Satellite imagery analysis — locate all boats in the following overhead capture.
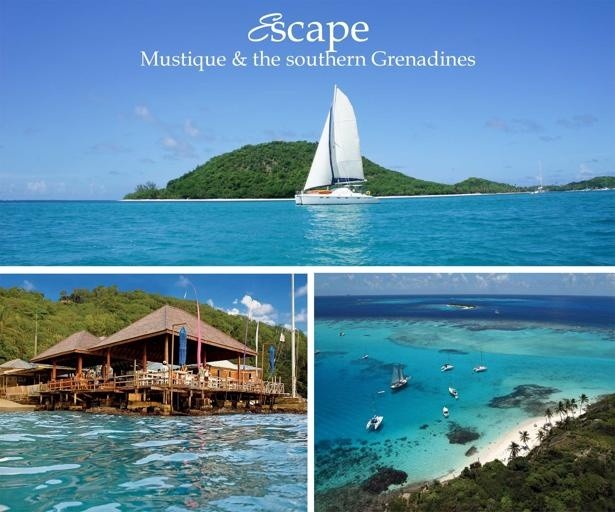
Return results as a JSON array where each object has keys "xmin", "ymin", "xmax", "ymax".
[{"xmin": 314, "ymin": 333, "xmax": 487, "ymax": 430}]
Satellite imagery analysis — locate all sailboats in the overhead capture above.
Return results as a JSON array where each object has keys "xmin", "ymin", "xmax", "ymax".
[{"xmin": 295, "ymin": 84, "xmax": 379, "ymax": 205}]
